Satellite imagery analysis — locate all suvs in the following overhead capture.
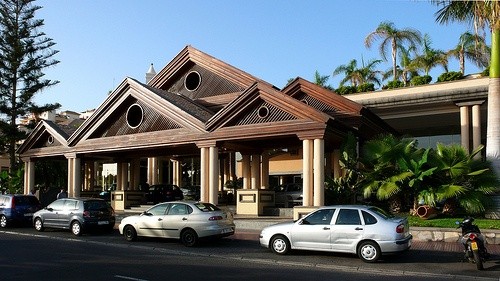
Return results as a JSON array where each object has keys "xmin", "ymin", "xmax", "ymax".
[
  {"xmin": 0, "ymin": 194, "xmax": 44, "ymax": 229},
  {"xmin": 32, "ymin": 197, "xmax": 116, "ymax": 236}
]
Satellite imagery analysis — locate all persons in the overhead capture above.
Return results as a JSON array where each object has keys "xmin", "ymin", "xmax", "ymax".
[
  {"xmin": 57, "ymin": 187, "xmax": 67, "ymax": 200},
  {"xmin": 0, "ymin": 189, "xmax": 7, "ymax": 196},
  {"xmin": 30, "ymin": 187, "xmax": 37, "ymax": 197}
]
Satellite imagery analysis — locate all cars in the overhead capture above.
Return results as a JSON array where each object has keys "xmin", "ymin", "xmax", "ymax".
[
  {"xmin": 259, "ymin": 204, "xmax": 413, "ymax": 263},
  {"xmin": 144, "ymin": 184, "xmax": 184, "ymax": 205},
  {"xmin": 118, "ymin": 200, "xmax": 236, "ymax": 248},
  {"xmin": 271, "ymin": 183, "xmax": 303, "ymax": 203},
  {"xmin": 99, "ymin": 184, "xmax": 117, "ymax": 200}
]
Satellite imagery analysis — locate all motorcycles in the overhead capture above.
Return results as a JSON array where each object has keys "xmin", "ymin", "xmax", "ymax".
[{"xmin": 455, "ymin": 216, "xmax": 488, "ymax": 270}]
